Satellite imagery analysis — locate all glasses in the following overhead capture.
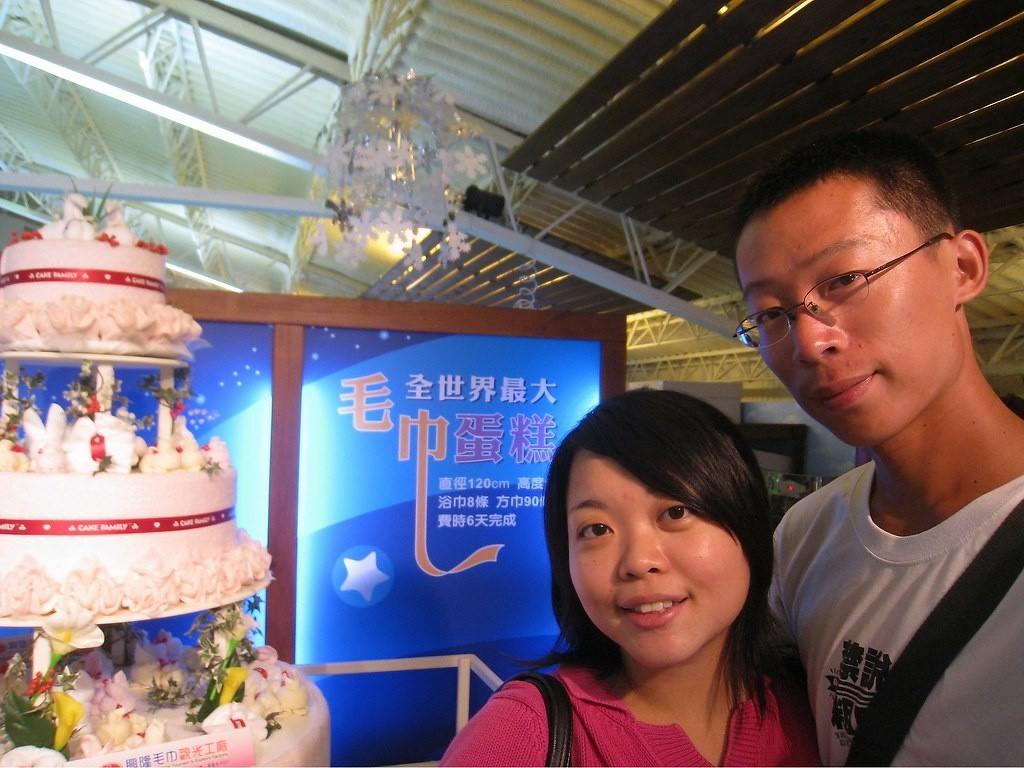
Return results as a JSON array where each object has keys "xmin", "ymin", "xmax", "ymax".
[{"xmin": 732, "ymin": 232, "xmax": 953, "ymax": 349}]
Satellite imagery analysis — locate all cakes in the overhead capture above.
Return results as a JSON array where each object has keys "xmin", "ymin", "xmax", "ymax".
[
  {"xmin": 0, "ymin": 598, "xmax": 331, "ymax": 768},
  {"xmin": 0, "ymin": 400, "xmax": 274, "ymax": 618},
  {"xmin": 0, "ymin": 180, "xmax": 203, "ymax": 360}
]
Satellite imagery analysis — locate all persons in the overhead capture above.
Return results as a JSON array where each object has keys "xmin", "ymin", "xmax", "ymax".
[
  {"xmin": 437, "ymin": 386, "xmax": 822, "ymax": 768},
  {"xmin": 732, "ymin": 141, "xmax": 1024, "ymax": 767}
]
